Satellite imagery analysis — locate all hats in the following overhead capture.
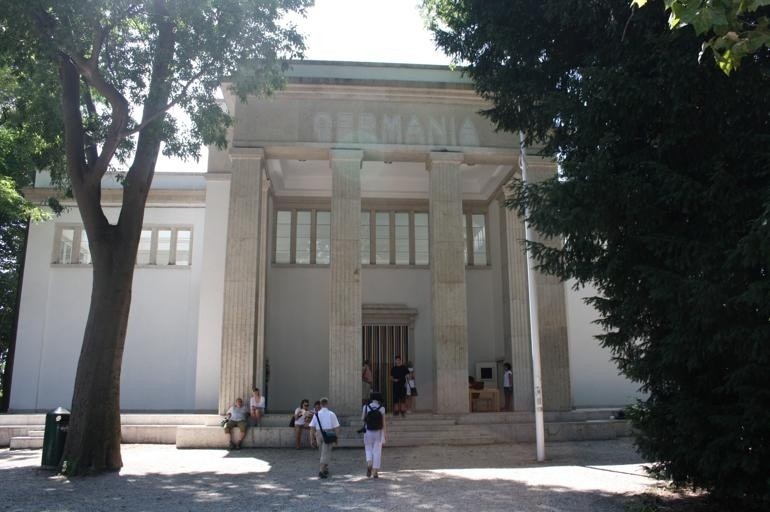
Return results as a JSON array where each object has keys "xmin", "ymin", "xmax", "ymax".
[{"xmin": 408, "ymin": 367, "xmax": 414, "ymax": 372}]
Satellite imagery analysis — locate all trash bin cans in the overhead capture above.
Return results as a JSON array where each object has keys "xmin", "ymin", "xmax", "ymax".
[{"xmin": 41, "ymin": 407, "xmax": 71, "ymax": 469}]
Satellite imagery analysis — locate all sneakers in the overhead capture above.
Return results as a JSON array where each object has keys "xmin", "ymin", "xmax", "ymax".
[
  {"xmin": 318, "ymin": 462, "xmax": 380, "ymax": 480},
  {"xmin": 229, "ymin": 443, "xmax": 241, "ymax": 450}
]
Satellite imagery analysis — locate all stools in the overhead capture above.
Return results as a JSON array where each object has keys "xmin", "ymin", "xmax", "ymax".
[{"xmin": 473, "ymin": 398, "xmax": 493, "ymax": 411}]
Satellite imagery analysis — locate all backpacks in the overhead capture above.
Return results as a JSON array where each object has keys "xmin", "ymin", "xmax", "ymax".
[{"xmin": 364, "ymin": 402, "xmax": 383, "ymax": 431}]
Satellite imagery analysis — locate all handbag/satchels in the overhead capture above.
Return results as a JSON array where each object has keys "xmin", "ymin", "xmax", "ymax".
[
  {"xmin": 410, "ymin": 387, "xmax": 417, "ymax": 396},
  {"xmin": 289, "ymin": 415, "xmax": 295, "ymax": 428},
  {"xmin": 323, "ymin": 429, "xmax": 337, "ymax": 445}
]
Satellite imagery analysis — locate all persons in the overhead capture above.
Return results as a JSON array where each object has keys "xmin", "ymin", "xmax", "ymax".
[
  {"xmin": 249, "ymin": 386, "xmax": 266, "ymax": 427},
  {"xmin": 310, "ymin": 396, "xmax": 341, "ymax": 478},
  {"xmin": 403, "ymin": 362, "xmax": 417, "ymax": 416},
  {"xmin": 308, "ymin": 400, "xmax": 320, "ymax": 450},
  {"xmin": 360, "ymin": 390, "xmax": 388, "ymax": 479},
  {"xmin": 388, "ymin": 354, "xmax": 409, "ymax": 418},
  {"xmin": 223, "ymin": 398, "xmax": 252, "ymax": 449},
  {"xmin": 609, "ymin": 410, "xmax": 626, "ymax": 420},
  {"xmin": 501, "ymin": 361, "xmax": 513, "ymax": 412},
  {"xmin": 363, "ymin": 359, "xmax": 374, "ymax": 404},
  {"xmin": 294, "ymin": 399, "xmax": 314, "ymax": 449}
]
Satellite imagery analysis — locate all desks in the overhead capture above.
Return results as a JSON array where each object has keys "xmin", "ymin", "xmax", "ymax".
[{"xmin": 469, "ymin": 386, "xmax": 501, "ymax": 412}]
